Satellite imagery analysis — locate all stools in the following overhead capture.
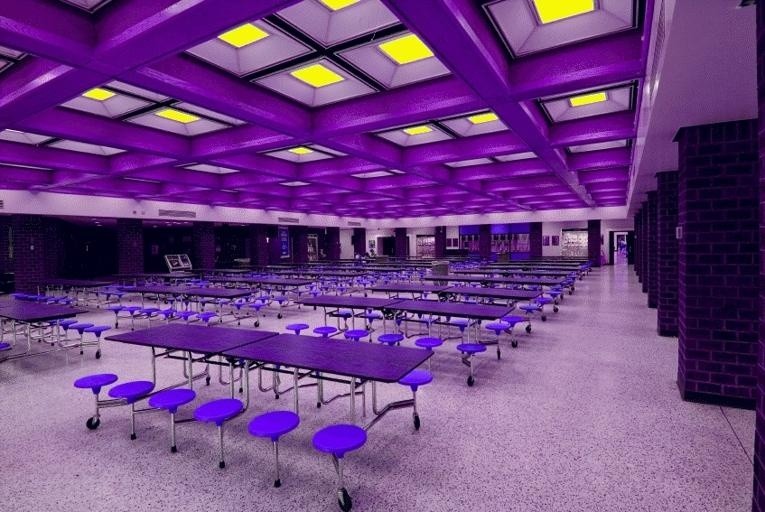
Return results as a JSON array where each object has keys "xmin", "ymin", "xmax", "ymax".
[
  {"xmin": 503, "ymin": 314, "xmax": 527, "ymax": 348},
  {"xmin": 107, "ymin": 380, "xmax": 155, "ymax": 441},
  {"xmin": 312, "ymin": 325, "xmax": 339, "ymax": 339},
  {"xmin": 10, "ymin": 257, "xmax": 314, "ymax": 327},
  {"xmin": 456, "ymin": 340, "xmax": 486, "ymax": 386},
  {"xmin": 396, "ymin": 370, "xmax": 431, "ymax": 431},
  {"xmin": 244, "ymin": 410, "xmax": 301, "ymax": 489},
  {"xmin": 193, "ymin": 396, "xmax": 244, "ymax": 471},
  {"xmin": 33, "ymin": 319, "xmax": 110, "ymax": 362},
  {"xmin": 147, "ymin": 386, "xmax": 196, "ymax": 454},
  {"xmin": 415, "ymin": 336, "xmax": 442, "ymax": 370},
  {"xmin": 311, "ymin": 424, "xmax": 368, "ymax": 511},
  {"xmin": 343, "ymin": 328, "xmax": 371, "ymax": 341},
  {"xmin": 377, "ymin": 333, "xmax": 406, "ymax": 347},
  {"xmin": 485, "ymin": 320, "xmax": 508, "ymax": 360},
  {"xmin": 301, "ymin": 255, "xmax": 596, "ymax": 333},
  {"xmin": 74, "ymin": 371, "xmax": 120, "ymax": 434},
  {"xmin": 285, "ymin": 322, "xmax": 311, "ymax": 334}
]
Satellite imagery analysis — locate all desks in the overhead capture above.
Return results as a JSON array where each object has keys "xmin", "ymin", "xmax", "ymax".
[
  {"xmin": 222, "ymin": 329, "xmax": 432, "ymax": 424},
  {"xmin": 0, "ymin": 297, "xmax": 91, "ymax": 355},
  {"xmin": 103, "ymin": 320, "xmax": 278, "ymax": 387}
]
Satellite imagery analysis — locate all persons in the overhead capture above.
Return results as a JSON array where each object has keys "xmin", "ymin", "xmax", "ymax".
[
  {"xmin": 620, "ymin": 237, "xmax": 627, "ymax": 256},
  {"xmin": 355, "ymin": 249, "xmax": 377, "ymax": 265}
]
